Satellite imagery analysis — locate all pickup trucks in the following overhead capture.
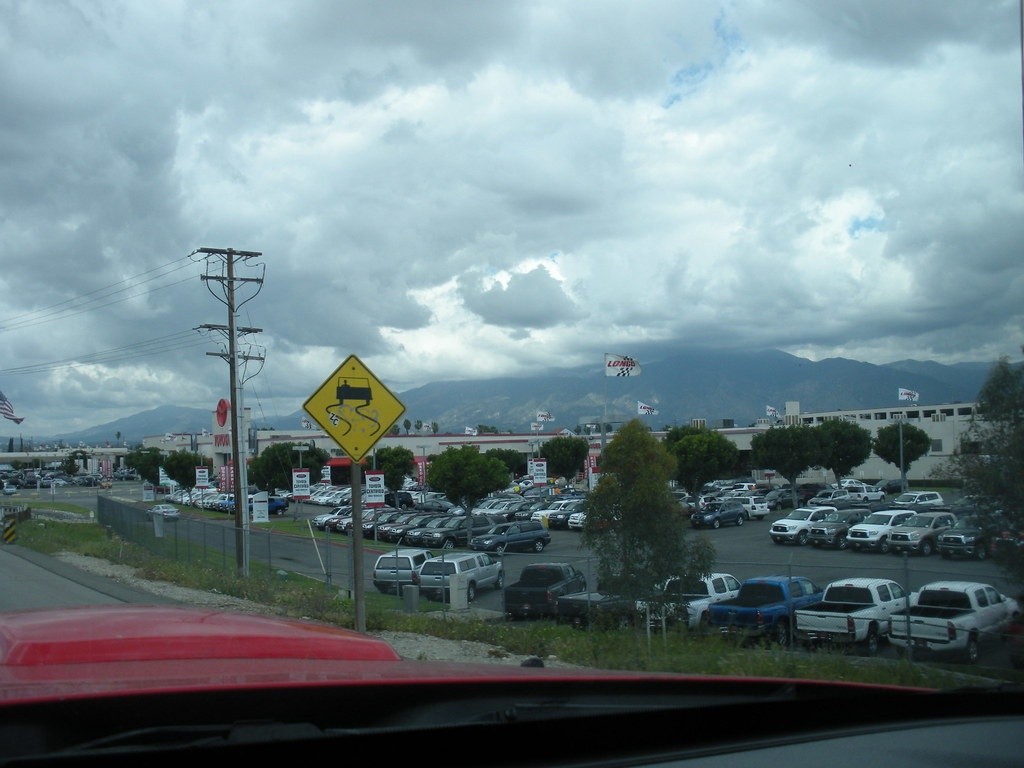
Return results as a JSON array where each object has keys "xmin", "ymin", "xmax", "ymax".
[
  {"xmin": 706, "ymin": 573, "xmax": 825, "ymax": 648},
  {"xmin": 793, "ymin": 576, "xmax": 919, "ymax": 658},
  {"xmin": 503, "ymin": 563, "xmax": 589, "ymax": 622},
  {"xmin": 553, "ymin": 563, "xmax": 680, "ymax": 633},
  {"xmin": 887, "ymin": 580, "xmax": 1023, "ymax": 665},
  {"xmin": 634, "ymin": 571, "xmax": 743, "ymax": 640}
]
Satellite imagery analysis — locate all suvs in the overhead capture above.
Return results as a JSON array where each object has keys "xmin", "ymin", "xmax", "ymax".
[
  {"xmin": 415, "ymin": 551, "xmax": 506, "ymax": 604},
  {"xmin": 371, "ymin": 548, "xmax": 435, "ymax": 596}
]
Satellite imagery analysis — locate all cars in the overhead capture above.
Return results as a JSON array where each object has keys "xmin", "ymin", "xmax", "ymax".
[
  {"xmin": 153, "ymin": 482, "xmax": 176, "ymax": 494},
  {"xmin": 2, "ymin": 485, "xmax": 17, "ymax": 495},
  {"xmin": 166, "ymin": 476, "xmax": 1024, "ymax": 563},
  {"xmin": 144, "ymin": 504, "xmax": 181, "ymax": 522},
  {"xmin": 9, "ymin": 462, "xmax": 140, "ymax": 489}
]
